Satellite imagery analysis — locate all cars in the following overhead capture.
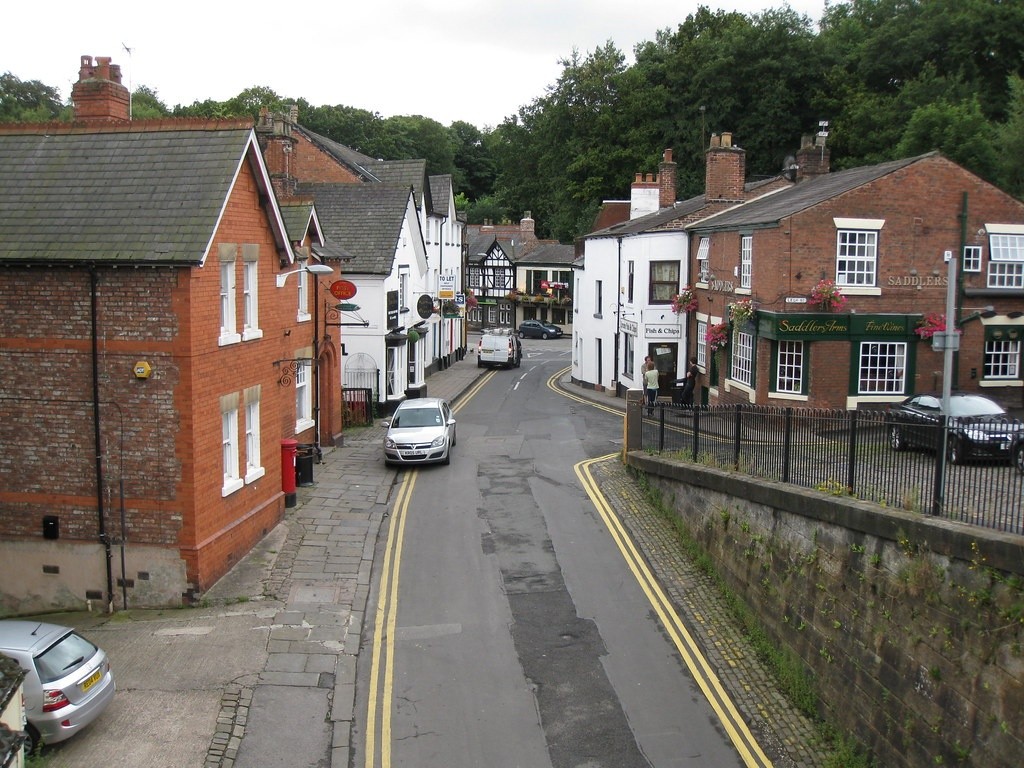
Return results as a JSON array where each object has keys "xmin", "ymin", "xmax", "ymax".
[
  {"xmin": 518, "ymin": 320, "xmax": 563, "ymax": 339},
  {"xmin": 1008, "ymin": 430, "xmax": 1024, "ymax": 475},
  {"xmin": 0, "ymin": 620, "xmax": 117, "ymax": 760},
  {"xmin": 886, "ymin": 389, "xmax": 1024, "ymax": 465},
  {"xmin": 380, "ymin": 397, "xmax": 456, "ymax": 466}
]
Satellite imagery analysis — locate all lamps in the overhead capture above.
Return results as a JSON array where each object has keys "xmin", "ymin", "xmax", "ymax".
[
  {"xmin": 276, "ymin": 265, "xmax": 334, "ymax": 288},
  {"xmin": 710, "ymin": 266, "xmax": 734, "ymax": 281},
  {"xmin": 704, "ymin": 266, "xmax": 724, "ymax": 281}
]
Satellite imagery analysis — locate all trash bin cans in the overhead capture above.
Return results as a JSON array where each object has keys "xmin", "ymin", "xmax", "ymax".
[
  {"xmin": 670, "ymin": 386, "xmax": 683, "ymax": 403},
  {"xmin": 296, "ymin": 443, "xmax": 314, "ymax": 488}
]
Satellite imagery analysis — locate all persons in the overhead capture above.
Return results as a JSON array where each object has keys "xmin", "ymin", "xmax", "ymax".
[
  {"xmin": 644, "ymin": 361, "xmax": 660, "ymax": 407},
  {"xmin": 681, "ymin": 357, "xmax": 698, "ymax": 408},
  {"xmin": 641, "ymin": 356, "xmax": 657, "ymax": 405}
]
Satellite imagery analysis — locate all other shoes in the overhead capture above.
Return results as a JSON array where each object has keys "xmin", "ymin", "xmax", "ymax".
[{"xmin": 648, "ymin": 413, "xmax": 653, "ymax": 416}]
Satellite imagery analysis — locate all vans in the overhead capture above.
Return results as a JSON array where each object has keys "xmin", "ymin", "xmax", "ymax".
[{"xmin": 476, "ymin": 329, "xmax": 522, "ymax": 368}]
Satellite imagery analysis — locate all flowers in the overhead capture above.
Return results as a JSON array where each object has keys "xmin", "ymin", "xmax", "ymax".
[
  {"xmin": 466, "ymin": 289, "xmax": 478, "ymax": 310},
  {"xmin": 810, "ymin": 278, "xmax": 849, "ymax": 312},
  {"xmin": 506, "ymin": 288, "xmax": 520, "ymax": 304},
  {"xmin": 443, "ymin": 299, "xmax": 460, "ymax": 314},
  {"xmin": 915, "ymin": 311, "xmax": 964, "ymax": 340},
  {"xmin": 705, "ymin": 322, "xmax": 728, "ymax": 373},
  {"xmin": 729, "ymin": 297, "xmax": 757, "ymax": 352},
  {"xmin": 672, "ymin": 285, "xmax": 699, "ymax": 316},
  {"xmin": 548, "ymin": 294, "xmax": 557, "ymax": 302}
]
